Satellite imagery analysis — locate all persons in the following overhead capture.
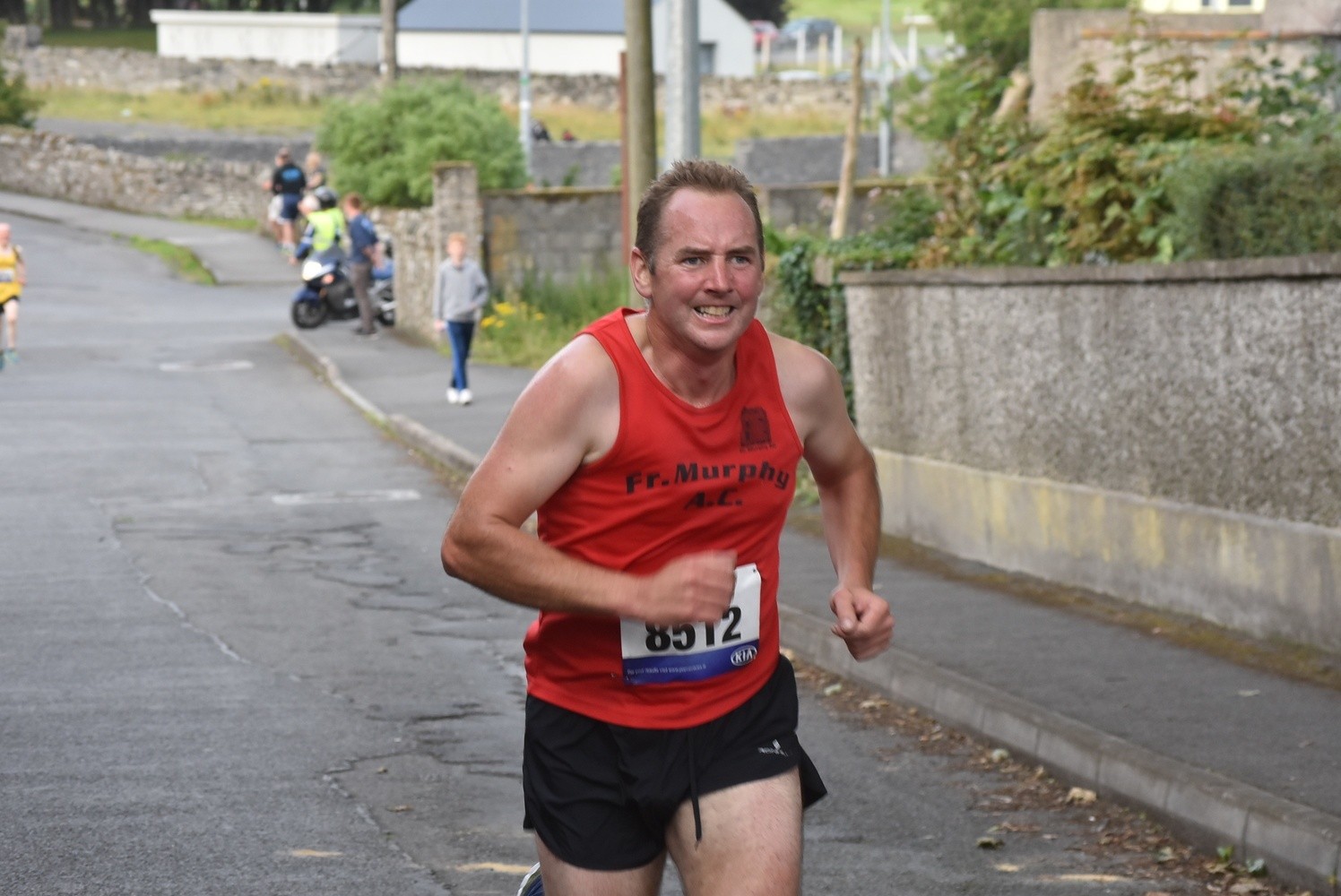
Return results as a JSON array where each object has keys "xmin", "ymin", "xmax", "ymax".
[
  {"xmin": 441, "ymin": 159, "xmax": 896, "ymax": 896},
  {"xmin": 0, "ymin": 222, "xmax": 27, "ymax": 355},
  {"xmin": 261, "ymin": 147, "xmax": 389, "ymax": 335},
  {"xmin": 434, "ymin": 232, "xmax": 489, "ymax": 408}
]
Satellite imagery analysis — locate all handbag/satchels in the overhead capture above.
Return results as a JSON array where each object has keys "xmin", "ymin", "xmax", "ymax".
[{"xmin": 267, "ymin": 194, "xmax": 284, "ymax": 220}]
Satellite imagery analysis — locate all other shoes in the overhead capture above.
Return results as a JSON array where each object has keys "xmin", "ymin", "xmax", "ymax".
[
  {"xmin": 459, "ymin": 389, "xmax": 473, "ymax": 405},
  {"xmin": 446, "ymin": 387, "xmax": 460, "ymax": 403}
]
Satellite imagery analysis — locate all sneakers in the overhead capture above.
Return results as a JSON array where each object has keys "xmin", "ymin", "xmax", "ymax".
[{"xmin": 517, "ymin": 861, "xmax": 546, "ymax": 896}]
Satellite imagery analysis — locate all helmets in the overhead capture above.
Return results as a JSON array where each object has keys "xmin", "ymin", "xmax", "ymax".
[{"xmin": 315, "ymin": 188, "xmax": 336, "ymax": 209}]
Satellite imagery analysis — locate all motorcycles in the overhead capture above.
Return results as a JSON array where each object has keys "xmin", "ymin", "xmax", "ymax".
[{"xmin": 290, "ymin": 243, "xmax": 395, "ymax": 330}]
